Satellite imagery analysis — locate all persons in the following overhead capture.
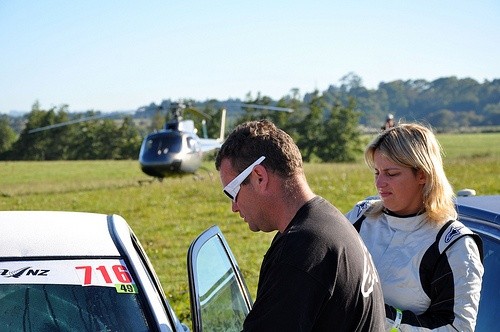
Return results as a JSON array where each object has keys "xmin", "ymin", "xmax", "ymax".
[
  {"xmin": 381, "ymin": 114, "xmax": 397, "ymax": 130},
  {"xmin": 215, "ymin": 120, "xmax": 387, "ymax": 332},
  {"xmin": 343, "ymin": 124, "xmax": 484, "ymax": 332}
]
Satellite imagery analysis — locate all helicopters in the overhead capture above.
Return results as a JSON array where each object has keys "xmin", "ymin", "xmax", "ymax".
[{"xmin": 27, "ymin": 99, "xmax": 294, "ymax": 184}]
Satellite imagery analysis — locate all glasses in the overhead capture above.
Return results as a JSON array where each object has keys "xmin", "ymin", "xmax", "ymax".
[{"xmin": 223, "ymin": 156, "xmax": 267, "ymax": 203}]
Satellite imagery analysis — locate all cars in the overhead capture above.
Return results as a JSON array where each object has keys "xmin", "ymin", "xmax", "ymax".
[
  {"xmin": 0, "ymin": 211, "xmax": 254, "ymax": 332},
  {"xmin": 369, "ymin": 195, "xmax": 500, "ymax": 332}
]
